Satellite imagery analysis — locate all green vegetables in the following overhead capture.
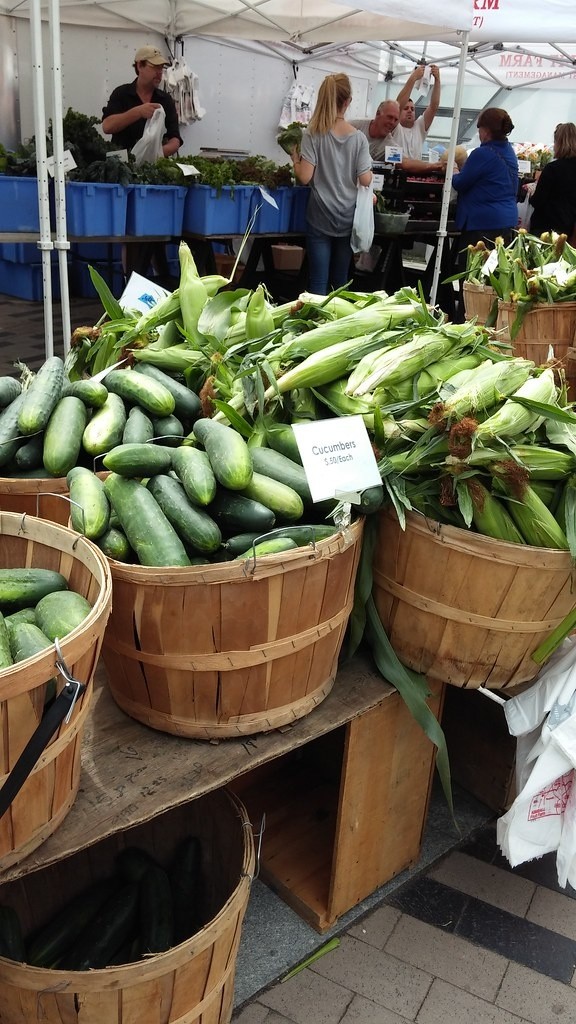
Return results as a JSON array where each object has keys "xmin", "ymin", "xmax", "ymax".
[{"xmin": 0, "ymin": 107, "xmax": 308, "ymax": 200}]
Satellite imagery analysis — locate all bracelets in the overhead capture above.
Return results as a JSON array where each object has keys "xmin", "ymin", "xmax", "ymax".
[{"xmin": 294, "ymin": 160, "xmax": 300, "ymax": 167}]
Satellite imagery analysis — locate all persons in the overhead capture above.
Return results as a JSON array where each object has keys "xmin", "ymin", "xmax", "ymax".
[
  {"xmin": 395, "ymin": 65, "xmax": 441, "ymax": 160},
  {"xmin": 347, "ymin": 99, "xmax": 447, "ymax": 273},
  {"xmin": 103, "ymin": 46, "xmax": 184, "ymax": 287},
  {"xmin": 528, "ymin": 123, "xmax": 576, "ymax": 247},
  {"xmin": 451, "ymin": 107, "xmax": 519, "ymax": 325},
  {"xmin": 289, "ymin": 73, "xmax": 373, "ymax": 301}
]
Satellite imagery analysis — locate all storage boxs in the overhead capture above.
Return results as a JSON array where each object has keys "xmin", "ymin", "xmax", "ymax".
[{"xmin": 0, "ymin": 175, "xmax": 311, "ymax": 302}]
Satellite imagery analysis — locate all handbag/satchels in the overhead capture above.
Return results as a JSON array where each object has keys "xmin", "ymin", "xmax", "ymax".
[
  {"xmin": 517, "ymin": 186, "xmax": 527, "ymax": 203},
  {"xmin": 515, "ymin": 191, "xmax": 534, "ymax": 234},
  {"xmin": 350, "ymin": 172, "xmax": 375, "ymax": 254}
]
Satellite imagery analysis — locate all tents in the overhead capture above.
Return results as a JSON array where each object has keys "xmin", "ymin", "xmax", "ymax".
[{"xmin": 1, "ymin": 0, "xmax": 576, "ymax": 361}]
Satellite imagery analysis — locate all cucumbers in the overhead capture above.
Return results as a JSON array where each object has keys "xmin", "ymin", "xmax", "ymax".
[{"xmin": 0, "ymin": 356, "xmax": 383, "ymax": 670}]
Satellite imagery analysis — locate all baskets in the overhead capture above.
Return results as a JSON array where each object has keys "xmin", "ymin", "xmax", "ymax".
[
  {"xmin": 375, "ymin": 501, "xmax": 576, "ymax": 691},
  {"xmin": 463, "ymin": 276, "xmax": 575, "ymax": 403},
  {"xmin": 2, "ymin": 470, "xmax": 118, "ymax": 528},
  {"xmin": 109, "ymin": 505, "xmax": 365, "ymax": 741},
  {"xmin": 1, "ymin": 515, "xmax": 113, "ymax": 867},
  {"xmin": 1, "ymin": 790, "xmax": 257, "ymax": 1024}
]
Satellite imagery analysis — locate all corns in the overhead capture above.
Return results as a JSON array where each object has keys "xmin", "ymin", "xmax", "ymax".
[{"xmin": 65, "ymin": 228, "xmax": 576, "ymax": 563}]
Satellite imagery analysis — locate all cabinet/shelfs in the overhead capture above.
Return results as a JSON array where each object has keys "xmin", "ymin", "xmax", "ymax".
[
  {"xmin": 233, "ymin": 673, "xmax": 448, "ymax": 935},
  {"xmin": 375, "ymin": 166, "xmax": 457, "ymax": 236}
]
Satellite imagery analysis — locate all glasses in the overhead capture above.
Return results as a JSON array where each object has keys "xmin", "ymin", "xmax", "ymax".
[{"xmin": 143, "ymin": 63, "xmax": 167, "ymax": 72}]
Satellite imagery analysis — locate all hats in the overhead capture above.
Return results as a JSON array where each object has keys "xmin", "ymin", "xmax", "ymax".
[
  {"xmin": 477, "ymin": 108, "xmax": 515, "ymax": 133},
  {"xmin": 134, "ymin": 46, "xmax": 172, "ymax": 67}
]
